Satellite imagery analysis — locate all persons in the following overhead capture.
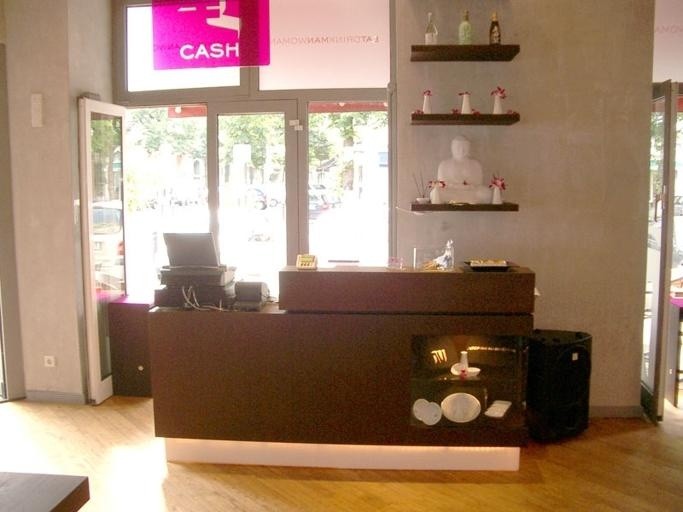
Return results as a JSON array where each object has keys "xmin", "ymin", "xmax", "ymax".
[{"xmin": 430, "ymin": 135, "xmax": 491, "ymax": 205}]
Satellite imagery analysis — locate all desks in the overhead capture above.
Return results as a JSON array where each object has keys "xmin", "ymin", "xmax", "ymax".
[{"xmin": 0, "ymin": 472, "xmax": 89, "ymax": 512}]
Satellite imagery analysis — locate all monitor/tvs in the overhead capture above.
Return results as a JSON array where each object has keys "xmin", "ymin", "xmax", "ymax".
[{"xmin": 162, "ymin": 232, "xmax": 218, "ymax": 267}]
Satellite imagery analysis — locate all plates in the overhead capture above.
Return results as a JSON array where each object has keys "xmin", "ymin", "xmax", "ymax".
[{"xmin": 470, "ymin": 256, "xmax": 509, "ymax": 272}]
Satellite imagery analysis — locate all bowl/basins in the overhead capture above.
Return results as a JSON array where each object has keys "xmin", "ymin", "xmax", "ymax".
[
  {"xmin": 467, "ymin": 367, "xmax": 480, "ymax": 376},
  {"xmin": 416, "ymin": 196, "xmax": 429, "ymax": 203},
  {"xmin": 450, "ymin": 362, "xmax": 460, "ymax": 375},
  {"xmin": 413, "ymin": 392, "xmax": 481, "ymax": 427}
]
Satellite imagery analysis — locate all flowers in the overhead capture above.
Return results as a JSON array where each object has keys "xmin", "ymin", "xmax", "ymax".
[
  {"xmin": 489, "ymin": 87, "xmax": 507, "ymax": 99},
  {"xmin": 489, "ymin": 175, "xmax": 506, "ymax": 191},
  {"xmin": 423, "ymin": 89, "xmax": 431, "ymax": 94},
  {"xmin": 458, "ymin": 90, "xmax": 471, "ymax": 95},
  {"xmin": 426, "ymin": 180, "xmax": 446, "ymax": 188}
]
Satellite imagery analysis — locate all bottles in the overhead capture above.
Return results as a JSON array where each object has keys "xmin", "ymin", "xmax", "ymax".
[
  {"xmin": 458, "ymin": 9, "xmax": 472, "ymax": 44},
  {"xmin": 489, "ymin": 10, "xmax": 501, "ymax": 45},
  {"xmin": 460, "ymin": 351, "xmax": 468, "ymax": 373},
  {"xmin": 425, "ymin": 12, "xmax": 439, "ymax": 44}
]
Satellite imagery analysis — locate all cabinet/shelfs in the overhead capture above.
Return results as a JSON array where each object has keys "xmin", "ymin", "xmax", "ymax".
[{"xmin": 411, "ymin": 44, "xmax": 521, "ymax": 212}]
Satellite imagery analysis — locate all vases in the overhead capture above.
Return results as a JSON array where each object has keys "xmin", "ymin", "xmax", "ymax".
[
  {"xmin": 461, "ymin": 96, "xmax": 471, "ymax": 115},
  {"xmin": 492, "ymin": 188, "xmax": 502, "ymax": 205},
  {"xmin": 492, "ymin": 97, "xmax": 503, "ymax": 114},
  {"xmin": 423, "ymin": 95, "xmax": 432, "ymax": 115},
  {"xmin": 430, "ymin": 188, "xmax": 440, "ymax": 204}
]
